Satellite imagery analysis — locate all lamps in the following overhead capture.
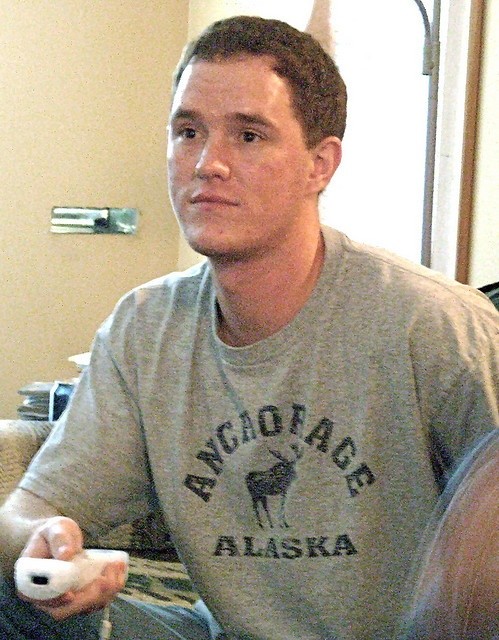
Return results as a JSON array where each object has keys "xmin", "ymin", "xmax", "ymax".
[{"xmin": 302, "ymin": 0, "xmax": 442, "ymax": 269}]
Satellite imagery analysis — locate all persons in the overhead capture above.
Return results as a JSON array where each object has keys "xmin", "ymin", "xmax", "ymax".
[{"xmin": 2, "ymin": 15, "xmax": 497, "ymax": 640}]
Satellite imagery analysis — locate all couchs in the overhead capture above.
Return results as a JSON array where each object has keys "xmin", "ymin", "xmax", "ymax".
[{"xmin": 0, "ymin": 420, "xmax": 222, "ymax": 640}]
